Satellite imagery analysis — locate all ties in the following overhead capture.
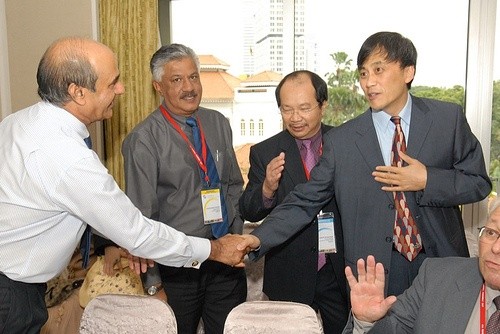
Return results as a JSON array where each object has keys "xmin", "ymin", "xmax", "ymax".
[
  {"xmin": 81, "ymin": 136, "xmax": 91, "ymax": 269},
  {"xmin": 303, "ymin": 139, "xmax": 326, "ymax": 272},
  {"xmin": 186, "ymin": 118, "xmax": 230, "ymax": 239},
  {"xmin": 485, "ymin": 295, "xmax": 500, "ymax": 334},
  {"xmin": 390, "ymin": 116, "xmax": 422, "ymax": 262}
]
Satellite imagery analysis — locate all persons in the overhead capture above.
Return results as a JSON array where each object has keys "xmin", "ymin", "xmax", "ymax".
[
  {"xmin": 237, "ymin": 69, "xmax": 349, "ymax": 333},
  {"xmin": 93, "ymin": 233, "xmax": 129, "ymax": 278},
  {"xmin": 120, "ymin": 43, "xmax": 246, "ymax": 334},
  {"xmin": 0, "ymin": 36, "xmax": 249, "ymax": 334},
  {"xmin": 233, "ymin": 31, "xmax": 493, "ymax": 317},
  {"xmin": 339, "ymin": 198, "xmax": 500, "ymax": 334}
]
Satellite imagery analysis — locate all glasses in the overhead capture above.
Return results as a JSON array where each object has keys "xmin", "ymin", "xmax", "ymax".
[
  {"xmin": 477, "ymin": 226, "xmax": 500, "ymax": 245},
  {"xmin": 280, "ymin": 103, "xmax": 319, "ymax": 114}
]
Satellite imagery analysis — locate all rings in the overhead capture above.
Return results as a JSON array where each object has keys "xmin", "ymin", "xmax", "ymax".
[{"xmin": 115, "ymin": 258, "xmax": 122, "ymax": 263}]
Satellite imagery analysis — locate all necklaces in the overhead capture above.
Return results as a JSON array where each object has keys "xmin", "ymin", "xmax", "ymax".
[{"xmin": 479, "ymin": 283, "xmax": 487, "ymax": 334}]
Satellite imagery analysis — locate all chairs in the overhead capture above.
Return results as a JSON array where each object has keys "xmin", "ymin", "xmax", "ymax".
[
  {"xmin": 79, "ymin": 293, "xmax": 177, "ymax": 334},
  {"xmin": 223, "ymin": 300, "xmax": 324, "ymax": 333}
]
